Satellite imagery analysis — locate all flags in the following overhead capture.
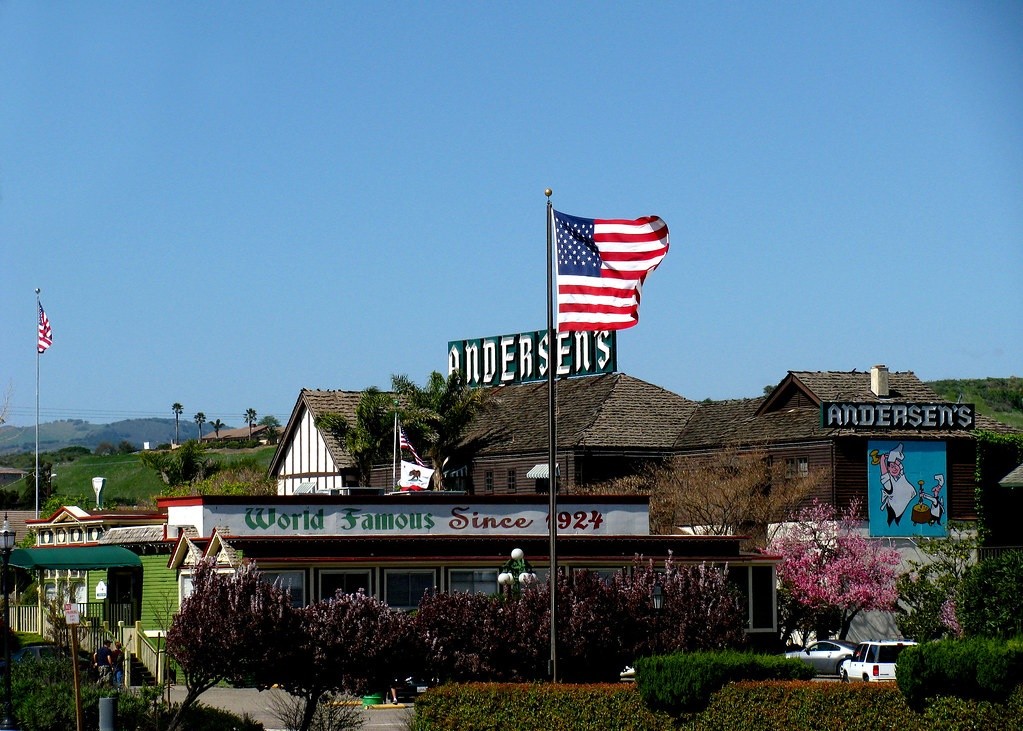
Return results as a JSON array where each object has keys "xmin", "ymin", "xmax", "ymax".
[
  {"xmin": 548, "ymin": 203, "xmax": 668, "ymax": 331},
  {"xmin": 400, "ymin": 426, "xmax": 424, "ymax": 467},
  {"xmin": 37, "ymin": 299, "xmax": 52, "ymax": 354}
]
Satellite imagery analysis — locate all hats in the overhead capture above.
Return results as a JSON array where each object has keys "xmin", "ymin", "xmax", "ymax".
[
  {"xmin": 116, "ymin": 642, "xmax": 121, "ymax": 647},
  {"xmin": 103, "ymin": 640, "xmax": 113, "ymax": 644}
]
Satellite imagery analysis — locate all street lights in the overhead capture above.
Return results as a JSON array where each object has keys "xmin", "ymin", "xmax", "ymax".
[
  {"xmin": 496, "ymin": 546, "xmax": 540, "ymax": 604},
  {"xmin": 0, "ymin": 511, "xmax": 29, "ymax": 731}
]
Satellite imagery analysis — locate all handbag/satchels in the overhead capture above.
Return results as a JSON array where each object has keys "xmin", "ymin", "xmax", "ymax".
[
  {"xmin": 109, "ymin": 671, "xmax": 113, "ymax": 685},
  {"xmin": 89, "ymin": 664, "xmax": 100, "ymax": 681},
  {"xmin": 116, "ymin": 652, "xmax": 124, "ymax": 668}
]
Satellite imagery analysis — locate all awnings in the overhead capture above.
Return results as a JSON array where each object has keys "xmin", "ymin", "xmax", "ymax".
[
  {"xmin": 526, "ymin": 463, "xmax": 559, "ymax": 479},
  {"xmin": 443, "ymin": 464, "xmax": 467, "ymax": 478}
]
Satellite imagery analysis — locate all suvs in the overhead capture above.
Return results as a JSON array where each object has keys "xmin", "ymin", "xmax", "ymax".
[{"xmin": 842, "ymin": 638, "xmax": 919, "ymax": 684}]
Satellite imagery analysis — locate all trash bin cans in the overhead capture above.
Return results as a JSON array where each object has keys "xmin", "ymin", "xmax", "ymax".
[{"xmin": 98, "ymin": 697, "xmax": 119, "ymax": 731}]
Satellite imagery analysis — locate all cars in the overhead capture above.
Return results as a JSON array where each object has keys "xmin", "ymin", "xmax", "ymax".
[
  {"xmin": 776, "ymin": 638, "xmax": 860, "ymax": 678},
  {"xmin": 0, "ymin": 644, "xmax": 90, "ymax": 684},
  {"xmin": 387, "ymin": 671, "xmax": 435, "ymax": 701}
]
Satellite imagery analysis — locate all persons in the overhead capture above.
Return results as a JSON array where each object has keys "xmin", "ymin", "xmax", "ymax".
[
  {"xmin": 93, "ymin": 640, "xmax": 124, "ymax": 688},
  {"xmin": 388, "ymin": 653, "xmax": 400, "ymax": 704}
]
